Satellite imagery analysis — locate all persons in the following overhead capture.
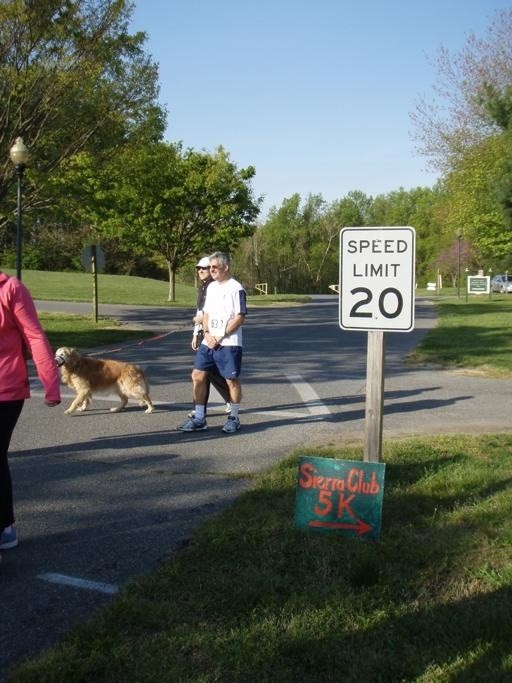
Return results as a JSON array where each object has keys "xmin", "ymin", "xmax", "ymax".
[
  {"xmin": 176, "ymin": 252, "xmax": 248, "ymax": 433},
  {"xmin": 187, "ymin": 256, "xmax": 232, "ymax": 418},
  {"xmin": 0, "ymin": 266, "xmax": 63, "ymax": 548}
]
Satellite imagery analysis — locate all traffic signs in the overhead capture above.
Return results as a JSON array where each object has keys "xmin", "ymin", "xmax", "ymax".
[{"xmin": 339, "ymin": 226, "xmax": 415, "ymax": 332}]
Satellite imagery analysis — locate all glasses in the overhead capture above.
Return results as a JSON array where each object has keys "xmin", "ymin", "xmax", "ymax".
[{"xmin": 198, "ymin": 267, "xmax": 207, "ymax": 270}]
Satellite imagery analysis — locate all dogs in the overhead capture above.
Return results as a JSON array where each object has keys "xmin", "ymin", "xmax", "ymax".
[{"xmin": 54, "ymin": 346, "xmax": 155, "ymax": 415}]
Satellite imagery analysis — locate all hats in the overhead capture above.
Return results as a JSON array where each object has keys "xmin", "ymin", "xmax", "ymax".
[{"xmin": 196, "ymin": 257, "xmax": 209, "ymax": 267}]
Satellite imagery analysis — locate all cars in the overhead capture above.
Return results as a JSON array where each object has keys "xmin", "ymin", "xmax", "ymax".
[{"xmin": 491, "ymin": 275, "xmax": 512, "ymax": 295}]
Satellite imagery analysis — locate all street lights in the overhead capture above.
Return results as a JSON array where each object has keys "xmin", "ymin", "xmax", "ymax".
[
  {"xmin": 9, "ymin": 137, "xmax": 26, "ymax": 283},
  {"xmin": 457, "ymin": 228, "xmax": 462, "ymax": 302}
]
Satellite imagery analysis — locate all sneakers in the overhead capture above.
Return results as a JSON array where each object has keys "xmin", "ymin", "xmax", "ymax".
[
  {"xmin": 0, "ymin": 525, "xmax": 17, "ymax": 549},
  {"xmin": 188, "ymin": 410, "xmax": 195, "ymax": 417},
  {"xmin": 178, "ymin": 418, "xmax": 208, "ymax": 432},
  {"xmin": 225, "ymin": 403, "xmax": 232, "ymax": 413},
  {"xmin": 222, "ymin": 416, "xmax": 241, "ymax": 432}
]
